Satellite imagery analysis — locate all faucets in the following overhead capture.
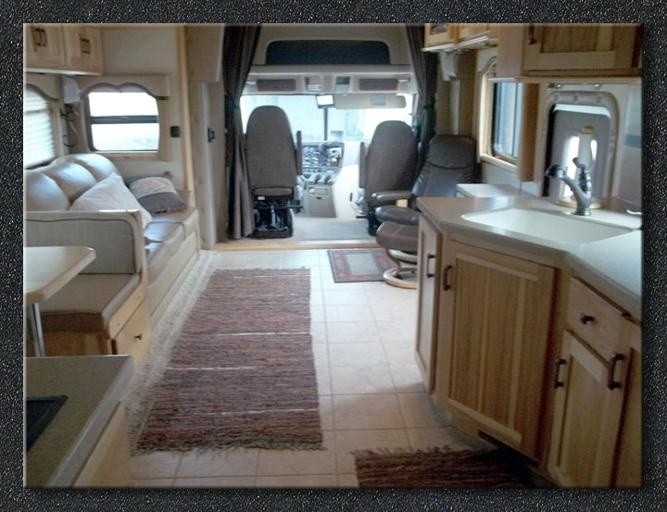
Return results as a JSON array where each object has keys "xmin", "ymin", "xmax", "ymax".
[{"xmin": 545, "ymin": 156, "xmax": 592, "ymax": 216}]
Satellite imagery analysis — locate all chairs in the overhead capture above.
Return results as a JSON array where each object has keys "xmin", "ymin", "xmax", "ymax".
[
  {"xmin": 241, "ymin": 105, "xmax": 303, "ymax": 239},
  {"xmin": 358, "ymin": 119, "xmax": 477, "ymax": 290}
]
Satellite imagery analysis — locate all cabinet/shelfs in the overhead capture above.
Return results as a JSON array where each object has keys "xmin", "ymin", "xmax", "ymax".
[
  {"xmin": 26, "ymin": 24, "xmax": 105, "ymax": 78},
  {"xmin": 422, "ymin": 24, "xmax": 498, "ymax": 48},
  {"xmin": 522, "ymin": 24, "xmax": 643, "ymax": 79},
  {"xmin": 26, "ymin": 282, "xmax": 151, "ymax": 370}
]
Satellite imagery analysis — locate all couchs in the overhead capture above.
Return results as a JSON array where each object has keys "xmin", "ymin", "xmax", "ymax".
[{"xmin": 22, "ymin": 154, "xmax": 202, "ymax": 265}]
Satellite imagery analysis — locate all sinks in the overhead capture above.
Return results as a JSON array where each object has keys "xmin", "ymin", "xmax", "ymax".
[{"xmin": 460, "ymin": 205, "xmax": 634, "ymax": 246}]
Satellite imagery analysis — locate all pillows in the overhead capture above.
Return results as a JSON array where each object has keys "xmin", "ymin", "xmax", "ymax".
[{"xmin": 69, "ymin": 171, "xmax": 187, "ymax": 230}]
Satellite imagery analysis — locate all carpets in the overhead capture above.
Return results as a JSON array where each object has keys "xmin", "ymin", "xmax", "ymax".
[
  {"xmin": 130, "ymin": 267, "xmax": 326, "ymax": 457},
  {"xmin": 327, "ymin": 243, "xmax": 385, "ymax": 282},
  {"xmin": 350, "ymin": 445, "xmax": 528, "ymax": 490}
]
